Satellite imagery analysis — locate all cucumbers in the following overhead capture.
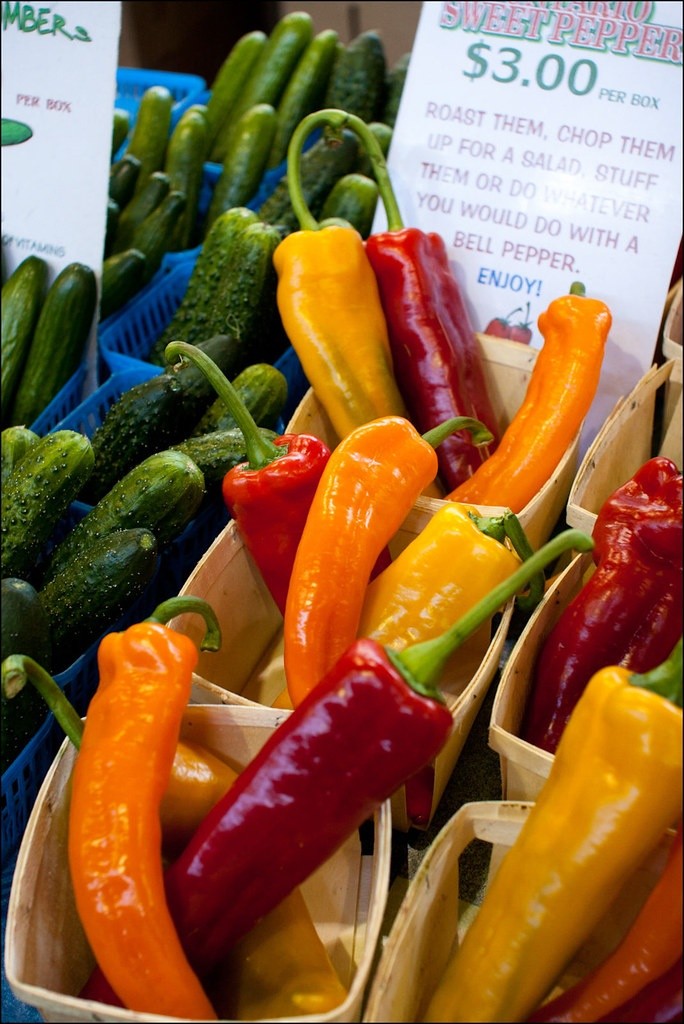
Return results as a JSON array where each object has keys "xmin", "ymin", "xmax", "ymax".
[{"xmin": 3, "ymin": 10, "xmax": 412, "ymax": 761}]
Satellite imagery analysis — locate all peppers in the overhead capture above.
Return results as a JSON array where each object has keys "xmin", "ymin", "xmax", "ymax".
[{"xmin": 1, "ymin": 109, "xmax": 684, "ymax": 1024}]
